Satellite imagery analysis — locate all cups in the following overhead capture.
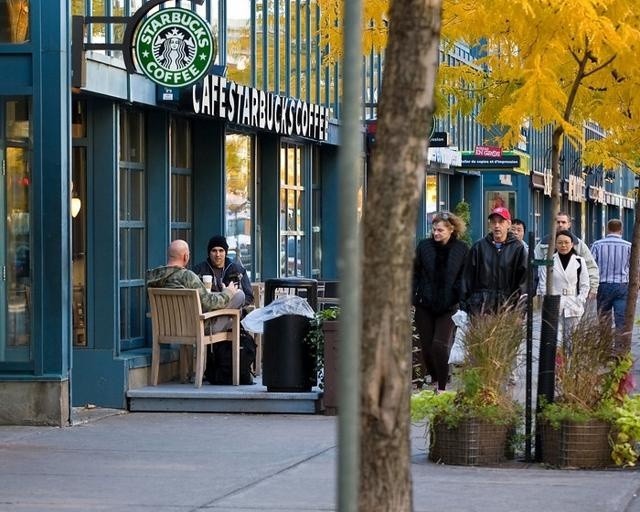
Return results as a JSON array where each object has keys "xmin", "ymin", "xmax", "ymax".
[{"xmin": 202, "ymin": 274, "xmax": 213, "ymax": 294}]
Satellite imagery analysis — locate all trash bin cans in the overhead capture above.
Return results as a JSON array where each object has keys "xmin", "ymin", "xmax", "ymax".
[{"xmin": 261, "ymin": 278, "xmax": 318, "ymax": 392}]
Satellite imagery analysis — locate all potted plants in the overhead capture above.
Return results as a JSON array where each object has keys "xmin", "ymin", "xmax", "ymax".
[
  {"xmin": 412, "ymin": 293, "xmax": 529, "ymax": 466},
  {"xmin": 538, "ymin": 303, "xmax": 640, "ymax": 470}
]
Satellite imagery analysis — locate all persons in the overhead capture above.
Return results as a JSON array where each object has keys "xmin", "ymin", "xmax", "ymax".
[
  {"xmin": 144, "ymin": 238, "xmax": 247, "ymax": 335},
  {"xmin": 507, "ymin": 218, "xmax": 529, "ymax": 386},
  {"xmin": 458, "ymin": 206, "xmax": 539, "ymax": 321},
  {"xmin": 192, "ymin": 235, "xmax": 256, "ymax": 381},
  {"xmin": 532, "ymin": 210, "xmax": 601, "ymax": 367},
  {"xmin": 589, "ymin": 218, "xmax": 633, "ymax": 358},
  {"xmin": 536, "ymin": 229, "xmax": 591, "ymax": 359},
  {"xmin": 409, "ymin": 210, "xmax": 472, "ymax": 390}
]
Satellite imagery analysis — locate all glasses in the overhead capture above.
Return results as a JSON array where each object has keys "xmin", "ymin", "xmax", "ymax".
[{"xmin": 437, "ymin": 211, "xmax": 453, "ymax": 224}]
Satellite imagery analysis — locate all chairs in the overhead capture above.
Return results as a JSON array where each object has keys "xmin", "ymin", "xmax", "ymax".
[{"xmin": 147, "ymin": 285, "xmax": 261, "ymax": 389}]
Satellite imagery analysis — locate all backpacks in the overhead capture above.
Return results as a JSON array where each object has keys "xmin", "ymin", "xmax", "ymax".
[{"xmin": 208, "ymin": 330, "xmax": 256, "ymax": 385}]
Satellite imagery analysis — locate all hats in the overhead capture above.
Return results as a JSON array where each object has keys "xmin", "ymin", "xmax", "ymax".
[
  {"xmin": 208, "ymin": 235, "xmax": 229, "ymax": 257},
  {"xmin": 488, "ymin": 207, "xmax": 511, "ymax": 221}
]
